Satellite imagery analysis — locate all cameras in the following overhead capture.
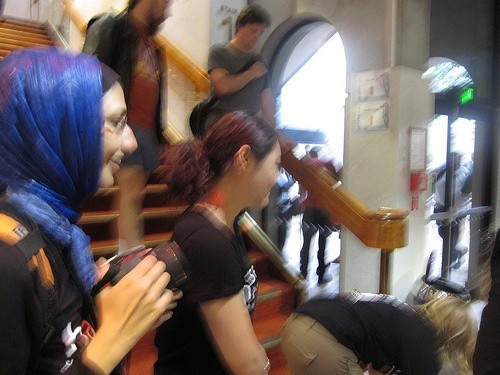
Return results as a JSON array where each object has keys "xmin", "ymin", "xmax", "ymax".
[{"xmin": 107, "ymin": 241, "xmax": 192, "ymax": 291}]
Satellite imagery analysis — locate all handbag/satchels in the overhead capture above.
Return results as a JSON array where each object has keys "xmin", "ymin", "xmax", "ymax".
[{"xmin": 189, "ymin": 97, "xmax": 208, "ymax": 140}]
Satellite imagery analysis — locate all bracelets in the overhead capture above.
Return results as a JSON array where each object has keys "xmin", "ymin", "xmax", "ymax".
[{"xmin": 264, "ymin": 359, "xmax": 270, "ymax": 372}]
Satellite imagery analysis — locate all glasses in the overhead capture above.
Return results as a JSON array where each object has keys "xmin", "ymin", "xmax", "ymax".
[{"xmin": 103, "ymin": 115, "xmax": 128, "ymax": 134}]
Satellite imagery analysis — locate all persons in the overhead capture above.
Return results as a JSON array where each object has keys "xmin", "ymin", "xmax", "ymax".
[
  {"xmin": 154, "ymin": 111, "xmax": 281, "ymax": 375},
  {"xmin": 0, "ymin": 46, "xmax": 172, "ymax": 375},
  {"xmin": 280, "ymin": 292, "xmax": 477, "ymax": 375},
  {"xmin": 1, "ymin": 0, "xmax": 500, "ymax": 375}
]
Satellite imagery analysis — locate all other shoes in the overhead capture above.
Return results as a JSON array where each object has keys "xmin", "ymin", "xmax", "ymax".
[{"xmin": 317, "ymin": 273, "xmax": 333, "ymax": 285}]
